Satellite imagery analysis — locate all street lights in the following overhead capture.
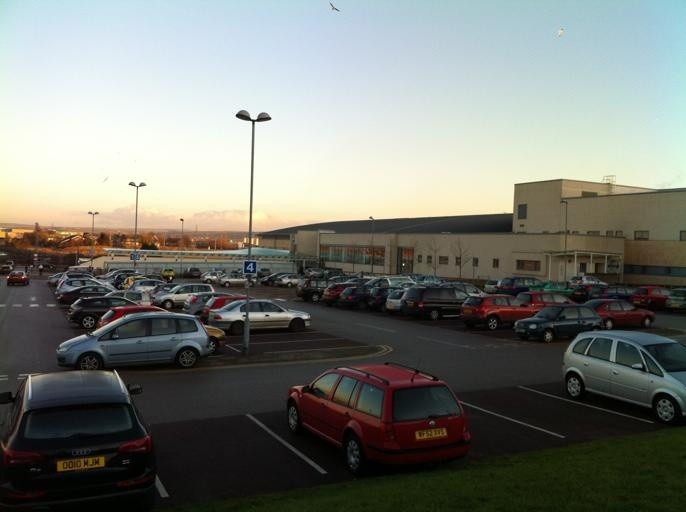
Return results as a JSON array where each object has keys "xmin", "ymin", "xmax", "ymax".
[
  {"xmin": 367, "ymin": 215, "xmax": 375, "ymax": 273},
  {"xmin": 559, "ymin": 199, "xmax": 570, "ymax": 283},
  {"xmin": 179, "ymin": 218, "xmax": 184, "ymax": 276},
  {"xmin": 234, "ymin": 109, "xmax": 272, "ymax": 354},
  {"xmin": 88, "ymin": 210, "xmax": 99, "ymax": 266},
  {"xmin": 128, "ymin": 180, "xmax": 147, "ymax": 285}
]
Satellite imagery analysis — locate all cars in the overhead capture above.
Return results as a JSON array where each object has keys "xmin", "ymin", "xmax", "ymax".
[
  {"xmin": 5, "ymin": 260, "xmax": 15, "ymax": 268},
  {"xmin": 561, "ymin": 327, "xmax": 686, "ymax": 427},
  {"xmin": 5, "ymin": 270, "xmax": 30, "ymax": 287},
  {"xmin": 0, "ymin": 265, "xmax": 12, "ymax": 274}
]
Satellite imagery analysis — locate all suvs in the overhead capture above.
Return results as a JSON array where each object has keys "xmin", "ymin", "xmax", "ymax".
[
  {"xmin": 0, "ymin": 366, "xmax": 160, "ymax": 511},
  {"xmin": 284, "ymin": 359, "xmax": 473, "ymax": 476}
]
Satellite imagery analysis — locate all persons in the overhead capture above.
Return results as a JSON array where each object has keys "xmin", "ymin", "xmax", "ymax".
[{"xmin": 39, "ymin": 264, "xmax": 44, "ymax": 276}]
